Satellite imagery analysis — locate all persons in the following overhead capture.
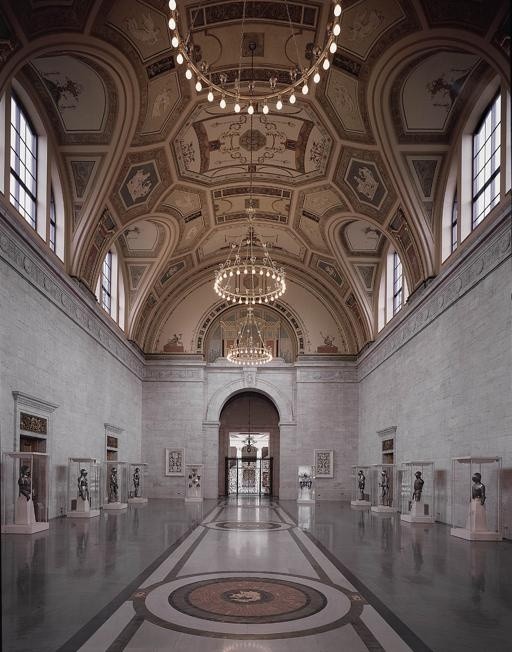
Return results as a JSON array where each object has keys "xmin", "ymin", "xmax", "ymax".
[
  {"xmin": 133, "ymin": 468, "xmax": 140, "ymax": 497},
  {"xmin": 378, "ymin": 470, "xmax": 390, "ymax": 506},
  {"xmin": 413, "ymin": 471, "xmax": 424, "ymax": 501},
  {"xmin": 78, "ymin": 468, "xmax": 89, "ymax": 501},
  {"xmin": 18, "ymin": 465, "xmax": 31, "ymax": 501},
  {"xmin": 109, "ymin": 468, "xmax": 118, "ymax": 502},
  {"xmin": 471, "ymin": 473, "xmax": 485, "ymax": 505},
  {"xmin": 358, "ymin": 470, "xmax": 365, "ymax": 499}
]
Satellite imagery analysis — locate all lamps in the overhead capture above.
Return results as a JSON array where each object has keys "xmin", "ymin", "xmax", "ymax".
[
  {"xmin": 211, "ymin": 201, "xmax": 288, "ymax": 369},
  {"xmin": 166, "ymin": 1, "xmax": 345, "ymax": 115}
]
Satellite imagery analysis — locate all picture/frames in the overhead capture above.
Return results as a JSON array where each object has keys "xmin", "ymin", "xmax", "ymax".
[
  {"xmin": 165, "ymin": 447, "xmax": 186, "ymax": 477},
  {"xmin": 314, "ymin": 448, "xmax": 334, "ymax": 478}
]
